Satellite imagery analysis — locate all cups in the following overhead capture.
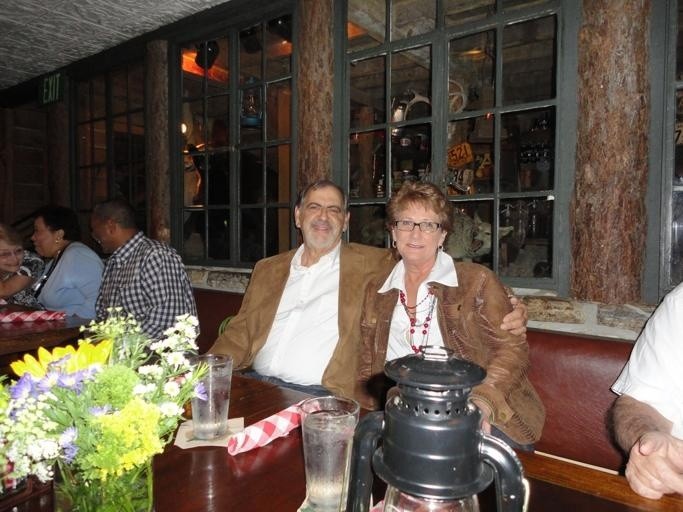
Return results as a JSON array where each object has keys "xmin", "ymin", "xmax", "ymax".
[
  {"xmin": 299, "ymin": 394, "xmax": 361, "ymax": 511},
  {"xmin": 186, "ymin": 353, "xmax": 234, "ymax": 440}
]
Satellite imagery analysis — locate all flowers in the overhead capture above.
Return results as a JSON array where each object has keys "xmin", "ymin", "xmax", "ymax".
[{"xmin": 0, "ymin": 301, "xmax": 211, "ymax": 493}]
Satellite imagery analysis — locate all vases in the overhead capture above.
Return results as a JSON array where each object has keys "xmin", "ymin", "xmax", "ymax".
[{"xmin": 50, "ymin": 452, "xmax": 157, "ymax": 512}]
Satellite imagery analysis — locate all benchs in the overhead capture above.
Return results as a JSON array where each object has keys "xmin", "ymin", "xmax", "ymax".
[{"xmin": 192, "ymin": 285, "xmax": 639, "ymax": 474}]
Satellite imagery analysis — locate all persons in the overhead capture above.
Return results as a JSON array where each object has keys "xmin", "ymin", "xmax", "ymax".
[
  {"xmin": 87, "ymin": 198, "xmax": 198, "ymax": 353},
  {"xmin": 27, "ymin": 205, "xmax": 104, "ymax": 324},
  {"xmin": 355, "ymin": 181, "xmax": 546, "ymax": 457},
  {"xmin": 605, "ymin": 277, "xmax": 681, "ymax": 503},
  {"xmin": 0, "ymin": 225, "xmax": 46, "ymax": 307},
  {"xmin": 199, "ymin": 177, "xmax": 529, "ymax": 409}
]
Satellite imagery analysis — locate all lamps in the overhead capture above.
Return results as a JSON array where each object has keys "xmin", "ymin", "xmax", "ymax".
[{"xmin": 192, "ymin": 13, "xmax": 296, "ymax": 73}]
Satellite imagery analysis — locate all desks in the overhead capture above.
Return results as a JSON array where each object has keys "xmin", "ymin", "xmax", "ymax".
[
  {"xmin": 0, "ymin": 373, "xmax": 683, "ymax": 512},
  {"xmin": 0, "ymin": 304, "xmax": 90, "ymax": 376}
]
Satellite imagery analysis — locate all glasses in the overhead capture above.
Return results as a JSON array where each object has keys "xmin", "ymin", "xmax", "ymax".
[{"xmin": 394, "ymin": 220, "xmax": 443, "ymax": 233}]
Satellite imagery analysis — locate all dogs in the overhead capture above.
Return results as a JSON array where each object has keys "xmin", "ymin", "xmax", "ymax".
[{"xmin": 444, "ymin": 203, "xmax": 515, "ymax": 260}]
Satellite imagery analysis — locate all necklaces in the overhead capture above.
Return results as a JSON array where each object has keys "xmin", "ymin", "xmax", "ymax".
[{"xmin": 397, "ymin": 285, "xmax": 437, "ymax": 361}]
